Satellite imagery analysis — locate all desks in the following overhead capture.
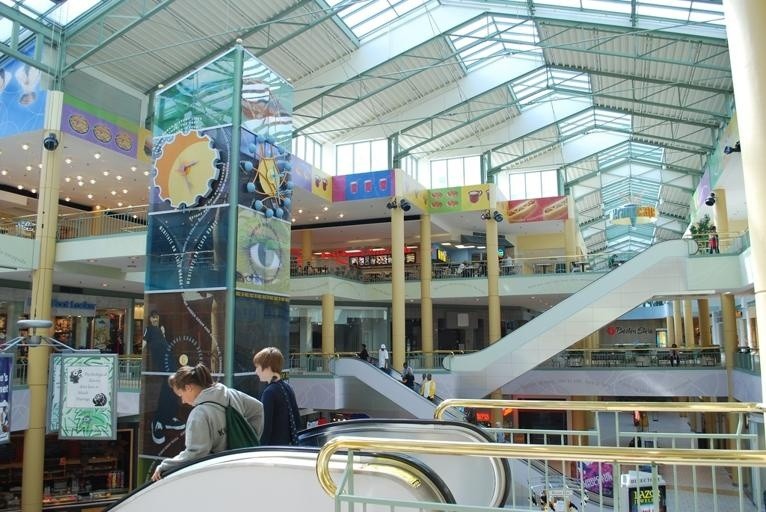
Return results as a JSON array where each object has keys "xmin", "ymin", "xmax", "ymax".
[
  {"xmin": 466, "ymin": 267, "xmax": 478, "ymax": 278},
  {"xmin": 502, "ymin": 264, "xmax": 515, "ymax": 276},
  {"xmin": 536, "ymin": 264, "xmax": 552, "ymax": 273},
  {"xmin": 576, "ymin": 261, "xmax": 590, "ymax": 272}
]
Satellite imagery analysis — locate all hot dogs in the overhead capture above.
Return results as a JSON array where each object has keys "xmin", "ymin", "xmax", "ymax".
[
  {"xmin": 507, "ymin": 200, "xmax": 538, "ymax": 221},
  {"xmin": 542, "ymin": 196, "xmax": 568, "ymax": 220}
]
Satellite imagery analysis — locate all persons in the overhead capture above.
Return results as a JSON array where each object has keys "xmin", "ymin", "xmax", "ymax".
[
  {"xmin": 708, "ymin": 226, "xmax": 720, "ymax": 254},
  {"xmin": 141, "ymin": 310, "xmax": 186, "ymax": 444},
  {"xmin": 252, "ymin": 347, "xmax": 301, "ymax": 447},
  {"xmin": 669, "ymin": 344, "xmax": 680, "ymax": 367},
  {"xmin": 432, "ymin": 254, "xmax": 516, "ymax": 280},
  {"xmin": 608, "ymin": 254, "xmax": 618, "ymax": 268},
  {"xmin": 357, "ymin": 344, "xmax": 435, "ymax": 402},
  {"xmin": 150, "ymin": 362, "xmax": 264, "ymax": 482}
]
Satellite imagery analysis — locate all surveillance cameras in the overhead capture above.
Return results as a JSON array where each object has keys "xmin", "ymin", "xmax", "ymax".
[
  {"xmin": 481, "ymin": 209, "xmax": 489, "ymax": 219},
  {"xmin": 400, "ymin": 202, "xmax": 411, "ymax": 211},
  {"xmin": 386, "ymin": 203, "xmax": 397, "ymax": 209},
  {"xmin": 724, "ymin": 145, "xmax": 732, "ymax": 154},
  {"xmin": 494, "ymin": 211, "xmax": 503, "ymax": 222},
  {"xmin": 43, "ymin": 137, "xmax": 58, "ymax": 151},
  {"xmin": 704, "ymin": 196, "xmax": 715, "ymax": 206}
]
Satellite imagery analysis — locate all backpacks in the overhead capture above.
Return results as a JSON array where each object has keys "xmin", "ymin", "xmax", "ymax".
[{"xmin": 194, "ymin": 398, "xmax": 260, "ymax": 450}]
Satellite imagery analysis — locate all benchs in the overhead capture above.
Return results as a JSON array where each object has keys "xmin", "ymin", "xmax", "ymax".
[
  {"xmin": 591, "ymin": 352, "xmax": 627, "ymax": 365},
  {"xmin": 656, "ymin": 351, "xmax": 696, "ymax": 367}
]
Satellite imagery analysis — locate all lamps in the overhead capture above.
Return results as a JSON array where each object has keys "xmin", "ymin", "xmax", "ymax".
[
  {"xmin": 723, "ymin": 142, "xmax": 740, "ymax": 155},
  {"xmin": 704, "ymin": 193, "xmax": 716, "ymax": 206},
  {"xmin": 386, "ymin": 198, "xmax": 410, "ymax": 211},
  {"xmin": 480, "ymin": 210, "xmax": 503, "ymax": 223}
]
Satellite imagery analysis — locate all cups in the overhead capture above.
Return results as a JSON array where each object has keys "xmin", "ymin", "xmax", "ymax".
[
  {"xmin": 350, "ymin": 182, "xmax": 358, "ymax": 195},
  {"xmin": 467, "ymin": 189, "xmax": 483, "ymax": 204},
  {"xmin": 364, "ymin": 179, "xmax": 372, "ymax": 193},
  {"xmin": 379, "ymin": 178, "xmax": 387, "ymax": 191}
]
